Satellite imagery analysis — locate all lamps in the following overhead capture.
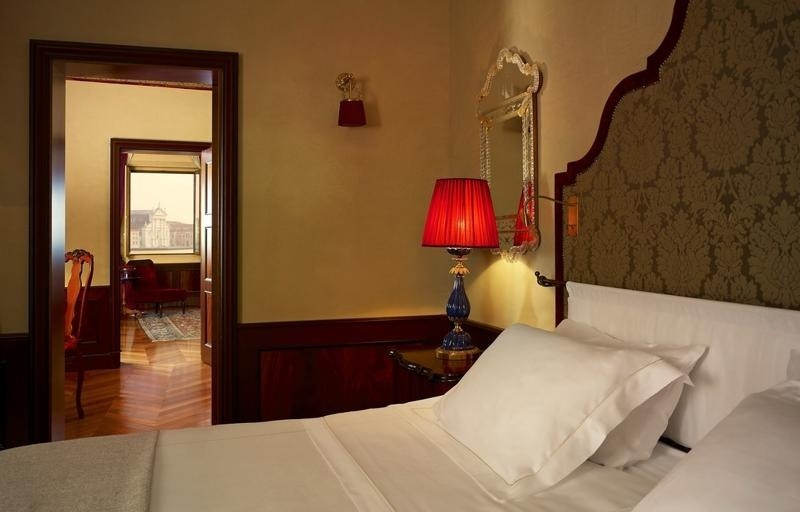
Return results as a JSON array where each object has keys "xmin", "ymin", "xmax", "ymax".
[
  {"xmin": 334, "ymin": 70, "xmax": 370, "ymax": 129},
  {"xmin": 419, "ymin": 176, "xmax": 503, "ymax": 361},
  {"xmin": 512, "ymin": 180, "xmax": 538, "ymax": 249}
]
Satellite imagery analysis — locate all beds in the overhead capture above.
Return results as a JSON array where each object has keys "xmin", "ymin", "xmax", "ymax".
[{"xmin": 0, "ymin": 0, "xmax": 800, "ymax": 512}]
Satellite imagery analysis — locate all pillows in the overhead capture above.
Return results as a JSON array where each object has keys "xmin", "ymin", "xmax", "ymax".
[
  {"xmin": 395, "ymin": 323, "xmax": 701, "ymax": 505},
  {"xmin": 549, "ymin": 316, "xmax": 710, "ymax": 474},
  {"xmin": 629, "ymin": 376, "xmax": 800, "ymax": 512}
]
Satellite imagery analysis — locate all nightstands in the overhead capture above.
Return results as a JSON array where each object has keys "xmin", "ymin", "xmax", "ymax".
[{"xmin": 387, "ymin": 343, "xmax": 482, "ymax": 402}]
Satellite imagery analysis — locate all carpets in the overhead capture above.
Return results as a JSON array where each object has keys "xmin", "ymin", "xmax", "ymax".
[{"xmin": 135, "ymin": 307, "xmax": 202, "ymax": 344}]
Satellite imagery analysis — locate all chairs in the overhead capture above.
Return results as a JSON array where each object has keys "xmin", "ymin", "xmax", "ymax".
[
  {"xmin": 63, "ymin": 247, "xmax": 96, "ymax": 420},
  {"xmin": 152, "ymin": 271, "xmax": 173, "ymax": 315},
  {"xmin": 178, "ymin": 268, "xmax": 201, "ymax": 307},
  {"xmin": 126, "ymin": 257, "xmax": 189, "ymax": 317}
]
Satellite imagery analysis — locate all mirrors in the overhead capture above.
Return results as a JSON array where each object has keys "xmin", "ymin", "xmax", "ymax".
[{"xmin": 471, "ymin": 45, "xmax": 543, "ymax": 266}]
